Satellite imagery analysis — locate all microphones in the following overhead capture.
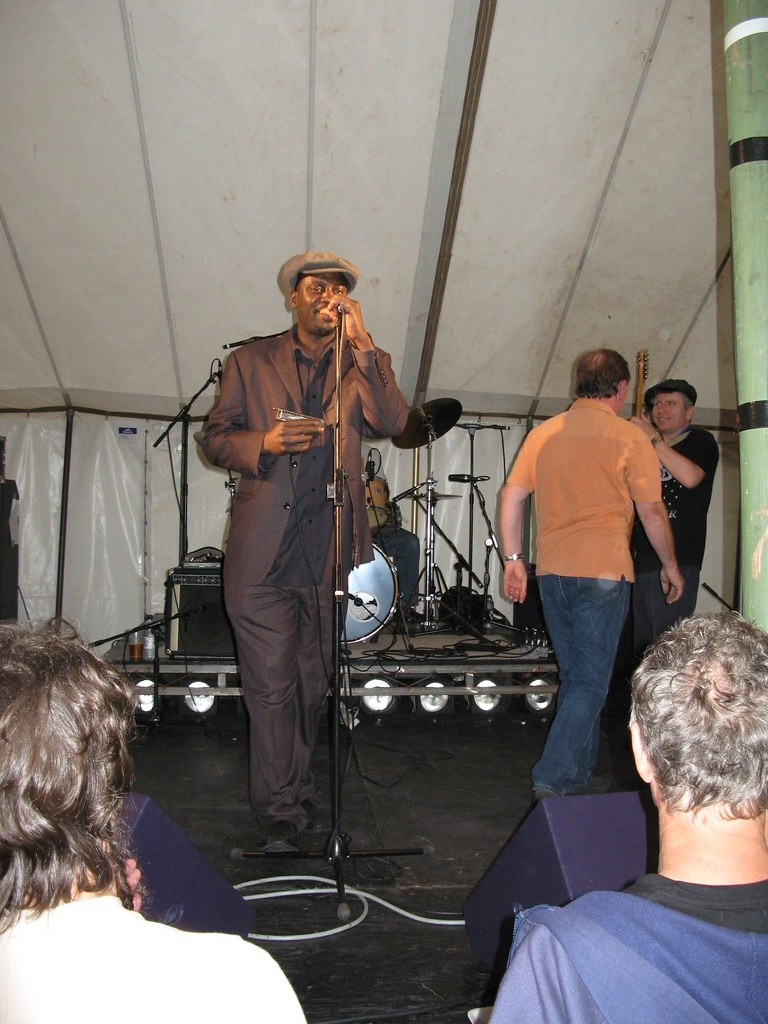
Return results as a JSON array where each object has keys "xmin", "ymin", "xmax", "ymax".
[
  {"xmin": 392, "ymin": 483, "xmax": 424, "ymax": 503},
  {"xmin": 217, "ymin": 362, "xmax": 223, "ymax": 387},
  {"xmin": 179, "ymin": 604, "xmax": 208, "ymax": 616},
  {"xmin": 448, "ymin": 474, "xmax": 486, "ymax": 483},
  {"xmin": 337, "ymin": 304, "xmax": 346, "ymax": 313},
  {"xmin": 365, "ymin": 449, "xmax": 373, "ymax": 473}
]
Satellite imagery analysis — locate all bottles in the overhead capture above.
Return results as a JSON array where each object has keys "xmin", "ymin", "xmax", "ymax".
[{"xmin": 143, "ymin": 628, "xmax": 155, "ymax": 661}]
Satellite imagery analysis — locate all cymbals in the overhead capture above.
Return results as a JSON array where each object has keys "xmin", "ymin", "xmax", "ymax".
[
  {"xmin": 391, "ymin": 397, "xmax": 463, "ymax": 450},
  {"xmin": 405, "ymin": 492, "xmax": 463, "ymax": 502}
]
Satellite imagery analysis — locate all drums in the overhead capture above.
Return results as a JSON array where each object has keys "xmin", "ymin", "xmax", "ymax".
[
  {"xmin": 335, "ymin": 542, "xmax": 400, "ymax": 644},
  {"xmin": 364, "ymin": 475, "xmax": 389, "ymax": 529}
]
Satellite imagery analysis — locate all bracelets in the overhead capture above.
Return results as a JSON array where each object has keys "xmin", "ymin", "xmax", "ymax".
[
  {"xmin": 651, "ymin": 435, "xmax": 662, "ymax": 448},
  {"xmin": 503, "ymin": 553, "xmax": 526, "ymax": 561}
]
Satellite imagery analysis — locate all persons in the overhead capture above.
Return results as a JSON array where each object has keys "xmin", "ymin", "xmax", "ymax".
[
  {"xmin": 500, "ymin": 347, "xmax": 684, "ymax": 799},
  {"xmin": 488, "ymin": 609, "xmax": 768, "ymax": 1024},
  {"xmin": 611, "ymin": 377, "xmax": 720, "ymax": 790},
  {"xmin": 0, "ymin": 616, "xmax": 308, "ymax": 1023},
  {"xmin": 199, "ymin": 252, "xmax": 408, "ymax": 854}
]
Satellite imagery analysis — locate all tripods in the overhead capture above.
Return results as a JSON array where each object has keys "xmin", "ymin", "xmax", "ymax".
[
  {"xmin": 88, "ymin": 611, "xmax": 208, "ymax": 743},
  {"xmin": 232, "ymin": 312, "xmax": 435, "ymax": 923},
  {"xmin": 370, "ymin": 422, "xmax": 529, "ymax": 654}
]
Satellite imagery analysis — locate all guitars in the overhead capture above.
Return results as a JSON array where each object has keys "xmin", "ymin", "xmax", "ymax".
[{"xmin": 631, "ymin": 349, "xmax": 649, "ymax": 564}]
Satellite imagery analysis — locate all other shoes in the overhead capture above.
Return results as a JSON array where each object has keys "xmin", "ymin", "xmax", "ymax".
[
  {"xmin": 302, "ymin": 797, "xmax": 343, "ymax": 827},
  {"xmin": 256, "ymin": 824, "xmax": 302, "ymax": 853},
  {"xmin": 534, "ymin": 787, "xmax": 564, "ymax": 801},
  {"xmin": 587, "ymin": 773, "xmax": 611, "ymax": 794}
]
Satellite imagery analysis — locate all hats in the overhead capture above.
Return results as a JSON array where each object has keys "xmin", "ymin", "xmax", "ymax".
[
  {"xmin": 643, "ymin": 379, "xmax": 698, "ymax": 406},
  {"xmin": 278, "ymin": 251, "xmax": 359, "ymax": 294}
]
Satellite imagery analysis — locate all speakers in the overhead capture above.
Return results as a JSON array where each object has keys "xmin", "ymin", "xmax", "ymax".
[
  {"xmin": 166, "ymin": 567, "xmax": 237, "ymax": 661},
  {"xmin": 513, "ymin": 564, "xmax": 551, "ymax": 645},
  {"xmin": 463, "ymin": 790, "xmax": 659, "ymax": 992},
  {"xmin": 114, "ymin": 789, "xmax": 256, "ymax": 941}
]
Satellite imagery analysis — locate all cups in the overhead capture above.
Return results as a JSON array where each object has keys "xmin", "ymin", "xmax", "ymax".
[{"xmin": 128, "ymin": 632, "xmax": 144, "ymax": 662}]
